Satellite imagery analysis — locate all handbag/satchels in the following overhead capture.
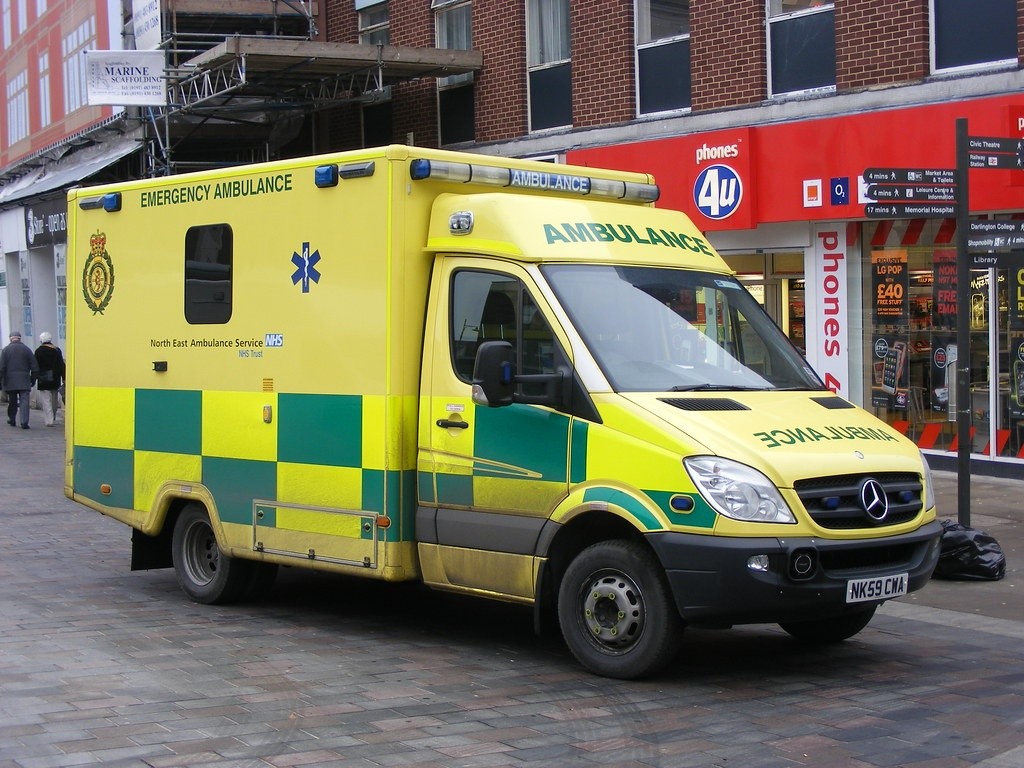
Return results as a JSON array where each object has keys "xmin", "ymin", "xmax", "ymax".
[{"xmin": 58, "ymin": 384, "xmax": 65, "ymax": 404}]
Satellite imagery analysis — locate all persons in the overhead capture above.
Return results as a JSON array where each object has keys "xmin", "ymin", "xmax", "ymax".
[
  {"xmin": 0, "ymin": 331, "xmax": 40, "ymax": 429},
  {"xmin": 31, "ymin": 332, "xmax": 66, "ymax": 427}
]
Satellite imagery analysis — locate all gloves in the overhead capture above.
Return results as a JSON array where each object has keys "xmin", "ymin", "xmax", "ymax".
[{"xmin": 29, "ymin": 377, "xmax": 36, "ymax": 387}]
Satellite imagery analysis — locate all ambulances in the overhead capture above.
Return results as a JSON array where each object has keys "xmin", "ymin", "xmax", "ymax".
[{"xmin": 63, "ymin": 144, "xmax": 944, "ymax": 684}]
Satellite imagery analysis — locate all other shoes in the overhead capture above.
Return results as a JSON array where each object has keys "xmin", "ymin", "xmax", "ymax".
[
  {"xmin": 6, "ymin": 419, "xmax": 16, "ymax": 426},
  {"xmin": 21, "ymin": 424, "xmax": 30, "ymax": 429}
]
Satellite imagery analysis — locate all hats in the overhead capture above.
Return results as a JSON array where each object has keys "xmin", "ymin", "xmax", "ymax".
[
  {"xmin": 40, "ymin": 332, "xmax": 52, "ymax": 343},
  {"xmin": 9, "ymin": 331, "xmax": 21, "ymax": 337}
]
